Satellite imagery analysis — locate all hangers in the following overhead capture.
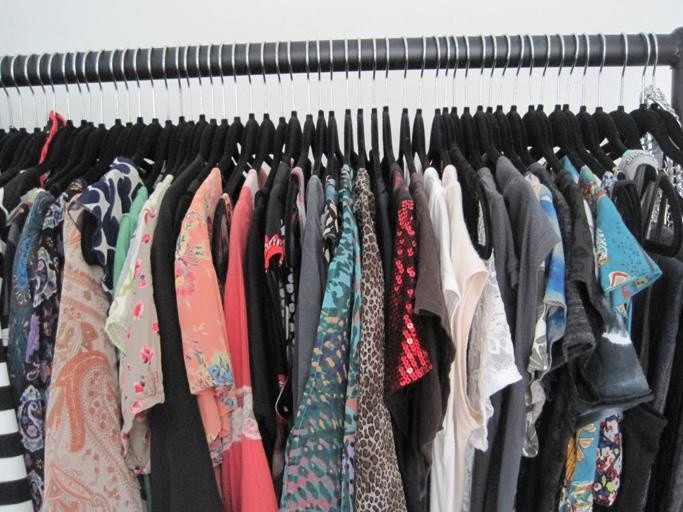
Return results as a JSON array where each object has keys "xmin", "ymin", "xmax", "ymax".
[{"xmin": 1, "ymin": 33, "xmax": 683, "ymax": 257}]
